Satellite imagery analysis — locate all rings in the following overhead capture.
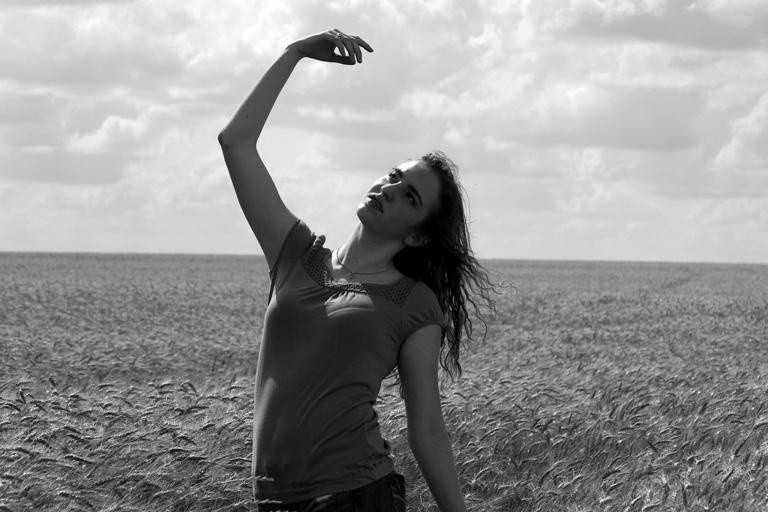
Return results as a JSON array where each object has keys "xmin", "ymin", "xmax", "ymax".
[{"xmin": 337, "ymin": 32, "xmax": 342, "ymax": 37}]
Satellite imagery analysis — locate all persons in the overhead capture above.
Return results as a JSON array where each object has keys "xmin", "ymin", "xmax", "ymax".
[{"xmin": 217, "ymin": 28, "xmax": 502, "ymax": 512}]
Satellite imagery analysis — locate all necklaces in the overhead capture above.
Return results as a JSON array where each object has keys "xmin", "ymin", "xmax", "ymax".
[{"xmin": 337, "ymin": 245, "xmax": 394, "ymax": 282}]
